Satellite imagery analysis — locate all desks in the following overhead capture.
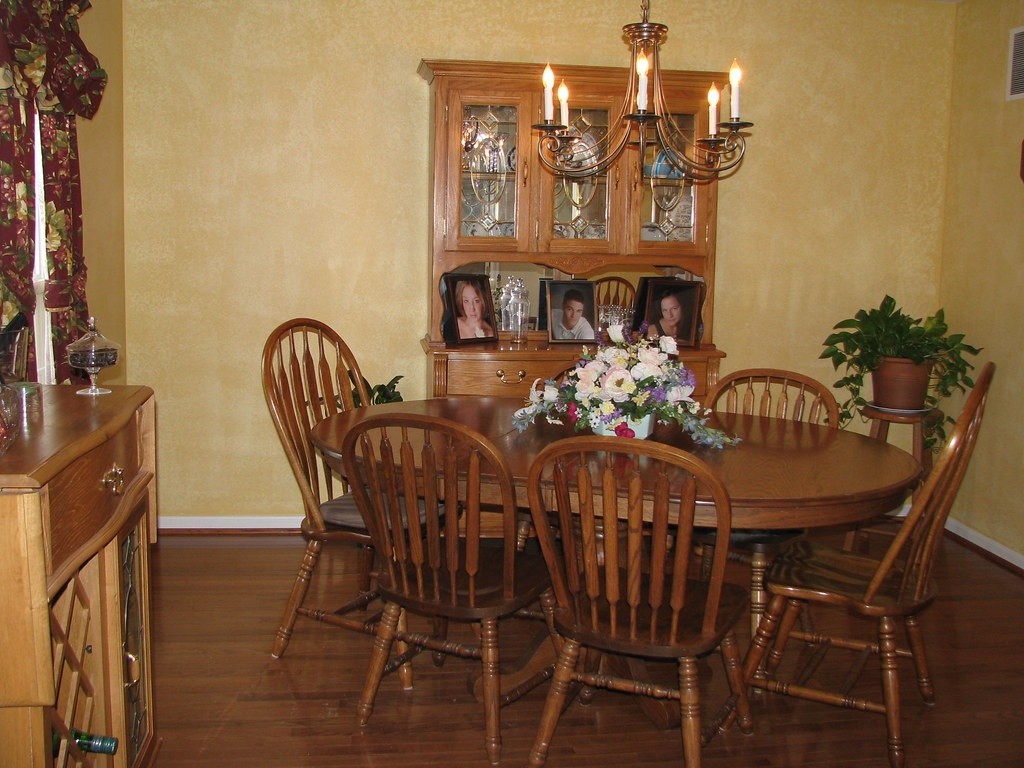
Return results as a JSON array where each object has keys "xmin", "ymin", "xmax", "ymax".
[
  {"xmin": 843, "ymin": 405, "xmax": 947, "ymax": 564},
  {"xmin": 309, "ymin": 395, "xmax": 922, "ymax": 730}
]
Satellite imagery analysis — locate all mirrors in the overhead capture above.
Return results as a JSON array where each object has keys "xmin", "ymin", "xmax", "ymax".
[{"xmin": 449, "ymin": 261, "xmax": 704, "ymax": 332}]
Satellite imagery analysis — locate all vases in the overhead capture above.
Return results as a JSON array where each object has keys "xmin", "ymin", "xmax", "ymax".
[{"xmin": 591, "ymin": 408, "xmax": 656, "ymax": 440}]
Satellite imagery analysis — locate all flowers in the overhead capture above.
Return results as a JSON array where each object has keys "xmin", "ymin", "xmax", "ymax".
[{"xmin": 511, "ymin": 321, "xmax": 743, "ymax": 450}]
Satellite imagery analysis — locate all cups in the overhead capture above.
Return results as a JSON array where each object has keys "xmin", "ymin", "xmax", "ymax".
[
  {"xmin": 0, "ymin": 325, "xmax": 29, "ymax": 384},
  {"xmin": 7, "ymin": 381, "xmax": 42, "ymax": 412}
]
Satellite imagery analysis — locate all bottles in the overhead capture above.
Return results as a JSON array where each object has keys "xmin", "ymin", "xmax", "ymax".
[
  {"xmin": 500, "ymin": 276, "xmax": 516, "ymax": 331},
  {"xmin": 508, "ymin": 278, "xmax": 530, "ymax": 343}
]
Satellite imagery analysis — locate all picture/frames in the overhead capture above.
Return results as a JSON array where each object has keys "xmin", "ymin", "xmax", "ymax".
[
  {"xmin": 645, "ymin": 280, "xmax": 703, "ymax": 347},
  {"xmin": 443, "ymin": 273, "xmax": 499, "ymax": 346},
  {"xmin": 545, "ymin": 279, "xmax": 598, "ymax": 344}
]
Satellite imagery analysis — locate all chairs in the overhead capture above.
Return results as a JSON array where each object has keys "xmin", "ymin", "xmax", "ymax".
[
  {"xmin": 595, "ymin": 277, "xmax": 635, "ymax": 322},
  {"xmin": 258, "ymin": 319, "xmax": 997, "ymax": 768}
]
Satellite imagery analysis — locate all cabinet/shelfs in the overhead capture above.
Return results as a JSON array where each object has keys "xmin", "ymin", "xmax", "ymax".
[
  {"xmin": 1, "ymin": 385, "xmax": 164, "ymax": 768},
  {"xmin": 416, "ymin": 58, "xmax": 726, "ymax": 536}
]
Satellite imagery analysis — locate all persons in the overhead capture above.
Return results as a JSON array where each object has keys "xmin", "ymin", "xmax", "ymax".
[
  {"xmin": 648, "ymin": 289, "xmax": 690, "ymax": 341},
  {"xmin": 455, "ymin": 280, "xmax": 495, "ymax": 339},
  {"xmin": 551, "ymin": 289, "xmax": 595, "ymax": 340}
]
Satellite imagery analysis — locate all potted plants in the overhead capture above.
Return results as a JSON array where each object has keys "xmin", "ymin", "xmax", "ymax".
[{"xmin": 818, "ymin": 293, "xmax": 985, "ymax": 454}]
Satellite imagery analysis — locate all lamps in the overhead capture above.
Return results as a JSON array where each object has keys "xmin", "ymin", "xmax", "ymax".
[{"xmin": 531, "ymin": 0, "xmax": 754, "ymax": 179}]
